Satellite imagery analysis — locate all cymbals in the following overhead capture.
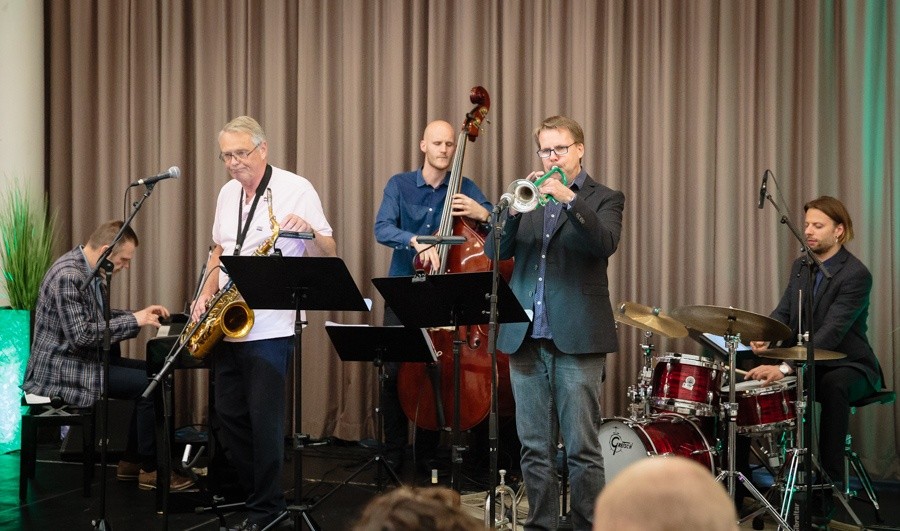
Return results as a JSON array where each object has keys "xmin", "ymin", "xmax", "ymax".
[
  {"xmin": 617, "ymin": 302, "xmax": 689, "ymax": 339},
  {"xmin": 756, "ymin": 344, "xmax": 847, "ymax": 361},
  {"xmin": 671, "ymin": 304, "xmax": 794, "ymax": 340},
  {"xmin": 613, "ymin": 312, "xmax": 669, "ymax": 337}
]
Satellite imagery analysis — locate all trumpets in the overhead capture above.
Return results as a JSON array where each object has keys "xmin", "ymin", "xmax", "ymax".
[{"xmin": 507, "ymin": 165, "xmax": 568, "ymax": 214}]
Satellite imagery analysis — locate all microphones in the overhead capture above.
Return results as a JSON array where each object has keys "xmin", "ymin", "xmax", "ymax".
[
  {"xmin": 757, "ymin": 170, "xmax": 768, "ymax": 210},
  {"xmin": 414, "ymin": 235, "xmax": 468, "ymax": 244},
  {"xmin": 492, "ymin": 192, "xmax": 515, "ymax": 214},
  {"xmin": 279, "ymin": 230, "xmax": 314, "ymax": 239},
  {"xmin": 130, "ymin": 166, "xmax": 180, "ymax": 187}
]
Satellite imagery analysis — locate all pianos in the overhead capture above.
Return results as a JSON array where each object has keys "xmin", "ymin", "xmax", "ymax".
[{"xmin": 146, "ymin": 313, "xmax": 209, "ymax": 369}]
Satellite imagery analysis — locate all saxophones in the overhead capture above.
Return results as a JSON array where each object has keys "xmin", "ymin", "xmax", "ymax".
[{"xmin": 180, "ymin": 188, "xmax": 281, "ymax": 363}]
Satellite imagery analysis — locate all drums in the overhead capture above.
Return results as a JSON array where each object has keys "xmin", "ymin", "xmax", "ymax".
[
  {"xmin": 599, "ymin": 411, "xmax": 715, "ymax": 488},
  {"xmin": 650, "ymin": 352, "xmax": 726, "ymax": 415},
  {"xmin": 720, "ymin": 376, "xmax": 798, "ymax": 433}
]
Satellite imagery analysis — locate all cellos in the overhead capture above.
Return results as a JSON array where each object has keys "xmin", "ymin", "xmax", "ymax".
[{"xmin": 395, "ymin": 86, "xmax": 517, "ymax": 485}]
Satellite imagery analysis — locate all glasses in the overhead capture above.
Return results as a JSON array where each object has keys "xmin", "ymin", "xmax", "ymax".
[
  {"xmin": 219, "ymin": 142, "xmax": 262, "ymax": 162},
  {"xmin": 537, "ymin": 141, "xmax": 578, "ymax": 158}
]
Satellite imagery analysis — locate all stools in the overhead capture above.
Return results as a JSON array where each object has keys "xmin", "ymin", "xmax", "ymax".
[{"xmin": 815, "ymin": 391, "xmax": 896, "ymax": 526}]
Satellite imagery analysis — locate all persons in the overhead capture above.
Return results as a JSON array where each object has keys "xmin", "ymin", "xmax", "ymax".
[
  {"xmin": 743, "ymin": 196, "xmax": 885, "ymax": 526},
  {"xmin": 373, "ymin": 120, "xmax": 493, "ymax": 480},
  {"xmin": 189, "ymin": 116, "xmax": 337, "ymax": 531},
  {"xmin": 484, "ymin": 116, "xmax": 625, "ymax": 531},
  {"xmin": 21, "ymin": 220, "xmax": 196, "ymax": 490},
  {"xmin": 350, "ymin": 454, "xmax": 741, "ymax": 531}
]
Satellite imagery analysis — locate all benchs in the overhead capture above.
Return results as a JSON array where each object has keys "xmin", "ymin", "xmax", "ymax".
[{"xmin": 20, "ymin": 397, "xmax": 97, "ymax": 504}]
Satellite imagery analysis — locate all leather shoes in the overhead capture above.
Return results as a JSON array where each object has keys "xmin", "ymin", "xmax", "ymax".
[
  {"xmin": 117, "ymin": 460, "xmax": 142, "ymax": 479},
  {"xmin": 138, "ymin": 468, "xmax": 194, "ymax": 492}
]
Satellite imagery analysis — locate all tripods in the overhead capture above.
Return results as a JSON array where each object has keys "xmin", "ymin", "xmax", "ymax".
[
  {"xmin": 219, "ymin": 255, "xmax": 368, "ymax": 531},
  {"xmin": 714, "ymin": 323, "xmax": 867, "ymax": 531},
  {"xmin": 323, "ymin": 322, "xmax": 435, "ymax": 489}
]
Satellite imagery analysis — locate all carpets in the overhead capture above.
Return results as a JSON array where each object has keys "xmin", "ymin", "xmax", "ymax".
[{"xmin": 457, "ymin": 485, "xmax": 878, "ymax": 531}]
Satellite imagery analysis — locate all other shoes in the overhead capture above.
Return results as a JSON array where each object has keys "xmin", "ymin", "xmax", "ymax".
[
  {"xmin": 382, "ymin": 463, "xmax": 401, "ymax": 482},
  {"xmin": 195, "ymin": 493, "xmax": 246, "ymax": 511},
  {"xmin": 249, "ymin": 502, "xmax": 286, "ymax": 525},
  {"xmin": 810, "ymin": 499, "xmax": 833, "ymax": 528},
  {"xmin": 424, "ymin": 460, "xmax": 450, "ymax": 476}
]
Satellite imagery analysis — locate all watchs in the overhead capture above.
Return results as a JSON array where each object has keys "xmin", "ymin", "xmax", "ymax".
[{"xmin": 779, "ymin": 364, "xmax": 790, "ymax": 377}]
